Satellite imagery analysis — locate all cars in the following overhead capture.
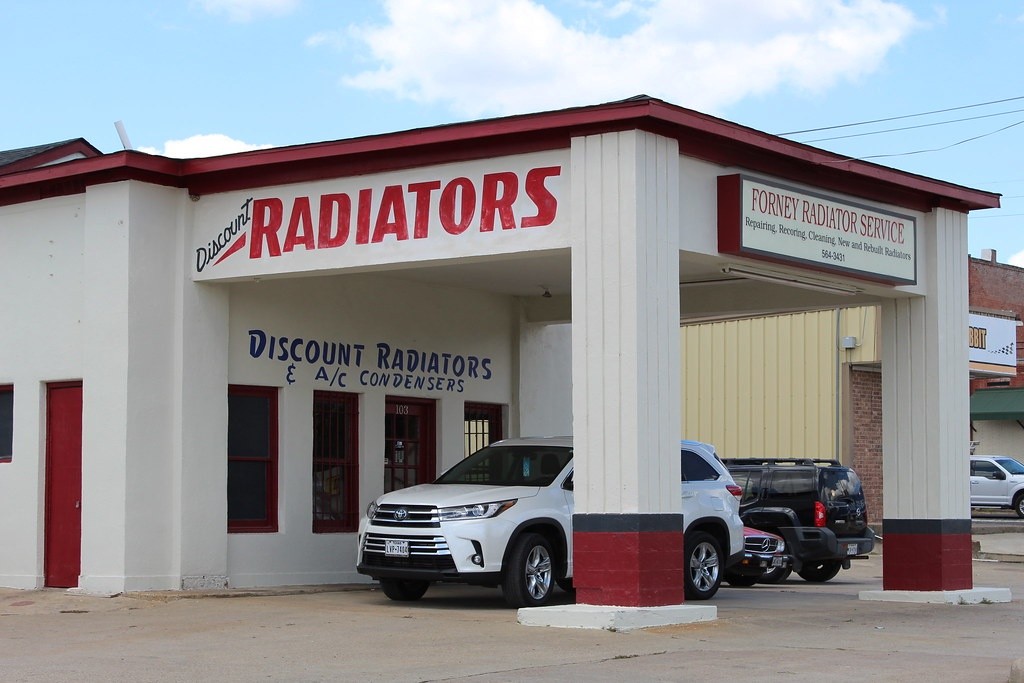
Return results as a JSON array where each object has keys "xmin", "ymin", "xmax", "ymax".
[
  {"xmin": 970, "ymin": 455, "xmax": 1024, "ymax": 519},
  {"xmin": 721, "ymin": 527, "xmax": 785, "ymax": 588}
]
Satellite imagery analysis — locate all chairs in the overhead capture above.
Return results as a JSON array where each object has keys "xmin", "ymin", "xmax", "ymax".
[{"xmin": 534, "ymin": 453, "xmax": 560, "ymax": 487}]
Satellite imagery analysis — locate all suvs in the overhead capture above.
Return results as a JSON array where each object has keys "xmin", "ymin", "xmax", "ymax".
[
  {"xmin": 357, "ymin": 437, "xmax": 746, "ymax": 610},
  {"xmin": 719, "ymin": 458, "xmax": 875, "ymax": 585}
]
